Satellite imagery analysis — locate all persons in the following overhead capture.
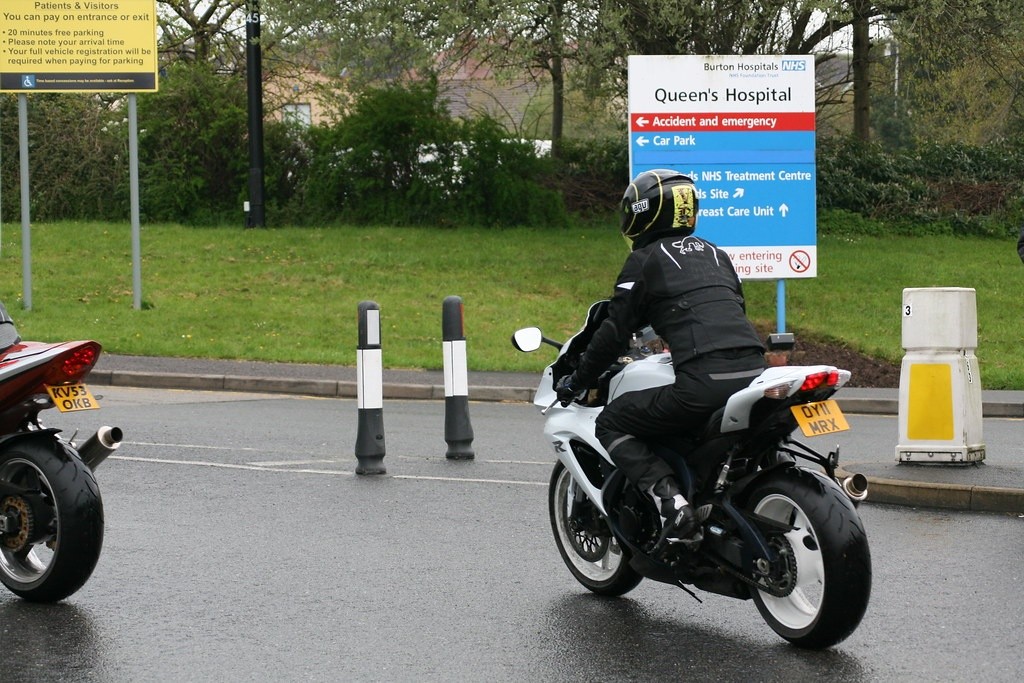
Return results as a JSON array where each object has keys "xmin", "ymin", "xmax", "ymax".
[{"xmin": 556, "ymin": 169, "xmax": 772, "ymax": 555}]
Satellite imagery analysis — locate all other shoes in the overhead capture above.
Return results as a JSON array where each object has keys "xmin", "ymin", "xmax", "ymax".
[{"xmin": 647, "ymin": 505, "xmax": 700, "ymax": 556}]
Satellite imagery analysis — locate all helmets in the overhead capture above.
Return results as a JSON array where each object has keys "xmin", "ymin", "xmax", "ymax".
[{"xmin": 619, "ymin": 168, "xmax": 700, "ymax": 252}]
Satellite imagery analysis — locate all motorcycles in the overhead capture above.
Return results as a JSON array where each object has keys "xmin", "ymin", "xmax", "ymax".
[
  {"xmin": 1, "ymin": 338, "xmax": 123, "ymax": 605},
  {"xmin": 511, "ymin": 300, "xmax": 873, "ymax": 651}
]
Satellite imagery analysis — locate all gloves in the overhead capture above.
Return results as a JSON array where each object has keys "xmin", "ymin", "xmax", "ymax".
[{"xmin": 556, "ymin": 374, "xmax": 579, "ymax": 403}]
